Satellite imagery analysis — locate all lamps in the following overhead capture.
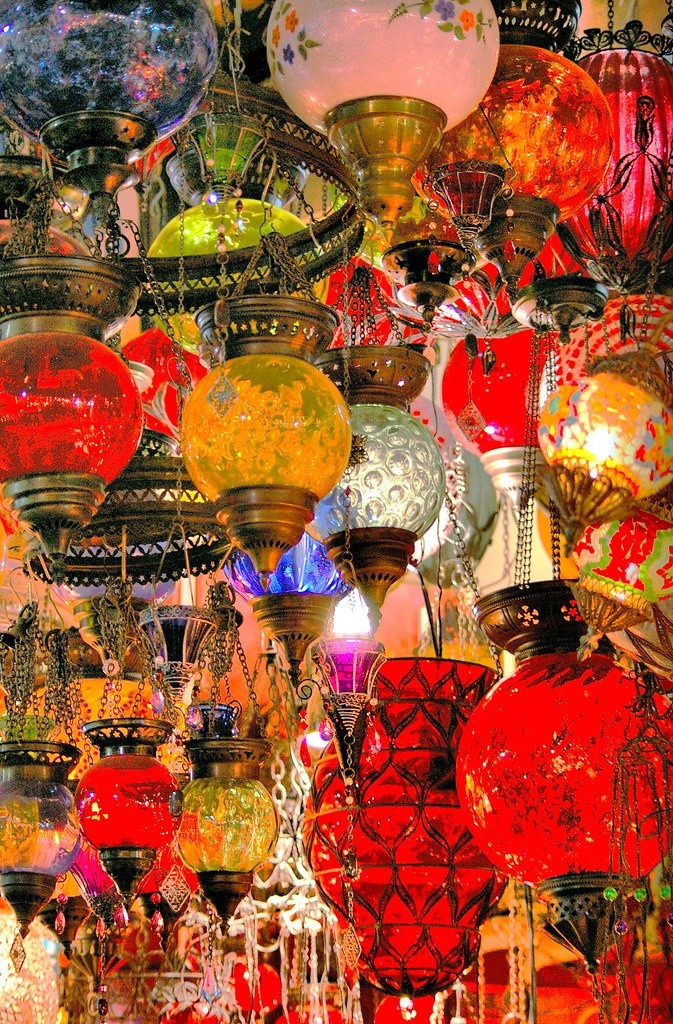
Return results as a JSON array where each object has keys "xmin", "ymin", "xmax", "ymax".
[{"xmin": 0, "ymin": 0, "xmax": 672, "ymax": 1024}]
